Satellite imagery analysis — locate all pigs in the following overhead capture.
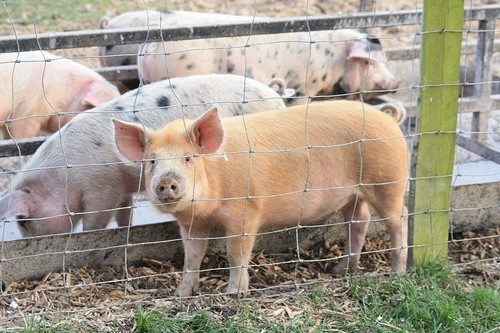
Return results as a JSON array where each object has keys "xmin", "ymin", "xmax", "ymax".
[
  {"xmin": 98, "ymin": 10, "xmax": 171, "ymax": 96},
  {"xmin": 111, "ymin": 100, "xmax": 411, "ymax": 298},
  {"xmin": 0, "ymin": 73, "xmax": 287, "ymax": 238},
  {"xmin": 137, "ymin": 10, "xmax": 402, "ymax": 107},
  {"xmin": 0, "ymin": 49, "xmax": 121, "ymax": 140}
]
